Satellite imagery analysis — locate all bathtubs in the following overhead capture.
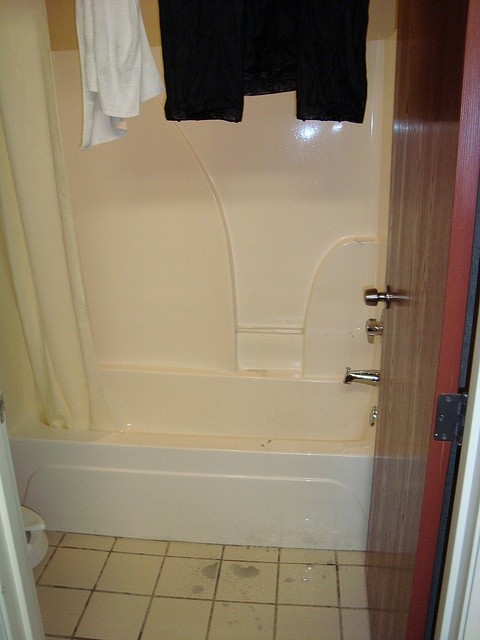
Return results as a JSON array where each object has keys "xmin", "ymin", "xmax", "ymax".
[{"xmin": 6, "ymin": 362, "xmax": 378, "ymax": 551}]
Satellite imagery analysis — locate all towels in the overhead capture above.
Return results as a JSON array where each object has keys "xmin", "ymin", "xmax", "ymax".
[{"xmin": 75, "ymin": 0, "xmax": 164, "ymax": 150}]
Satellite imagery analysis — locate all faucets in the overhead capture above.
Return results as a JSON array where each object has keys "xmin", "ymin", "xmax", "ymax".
[{"xmin": 343, "ymin": 367, "xmax": 380, "ymax": 387}]
